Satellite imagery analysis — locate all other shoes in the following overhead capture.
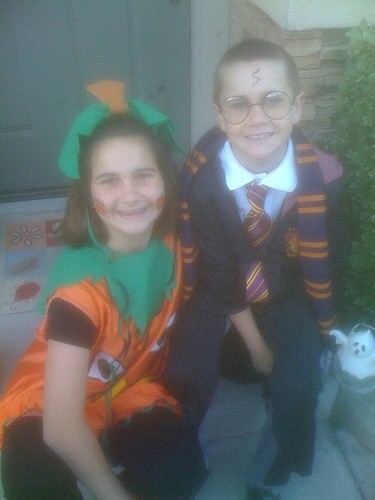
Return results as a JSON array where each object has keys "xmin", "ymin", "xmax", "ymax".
[{"xmin": 247, "ymin": 444, "xmax": 291, "ymax": 500}]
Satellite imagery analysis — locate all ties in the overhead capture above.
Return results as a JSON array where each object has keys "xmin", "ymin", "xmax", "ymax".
[{"xmin": 242, "ymin": 181, "xmax": 271, "ymax": 304}]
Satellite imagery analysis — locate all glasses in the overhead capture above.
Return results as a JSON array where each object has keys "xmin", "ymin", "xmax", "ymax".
[{"xmin": 219, "ymin": 90, "xmax": 297, "ymax": 124}]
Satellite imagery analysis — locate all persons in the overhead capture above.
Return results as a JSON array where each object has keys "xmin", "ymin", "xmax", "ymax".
[
  {"xmin": 0, "ymin": 77, "xmax": 206, "ymax": 500},
  {"xmin": 158, "ymin": 38, "xmax": 353, "ymax": 500}
]
